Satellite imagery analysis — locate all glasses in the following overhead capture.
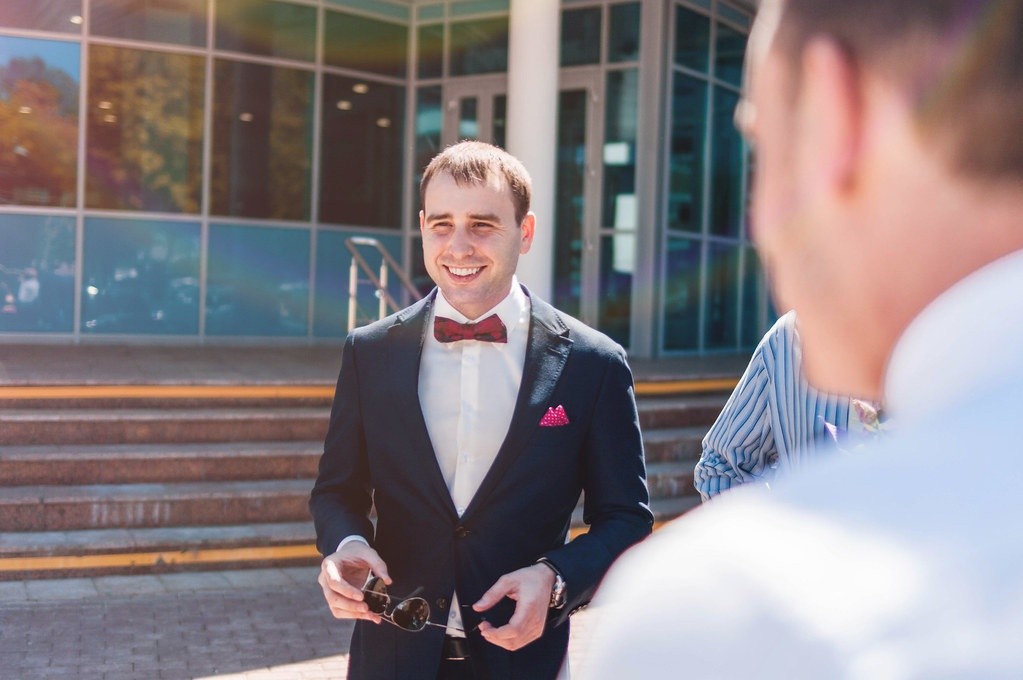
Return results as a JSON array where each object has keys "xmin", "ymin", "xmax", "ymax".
[{"xmin": 363, "ymin": 577, "xmax": 482, "ymax": 633}]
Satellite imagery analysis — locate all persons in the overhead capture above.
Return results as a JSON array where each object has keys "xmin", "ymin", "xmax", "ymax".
[
  {"xmin": 694, "ymin": 309, "xmax": 891, "ymax": 508},
  {"xmin": 310, "ymin": 141, "xmax": 655, "ymax": 680},
  {"xmin": 555, "ymin": 0, "xmax": 1022, "ymax": 680}
]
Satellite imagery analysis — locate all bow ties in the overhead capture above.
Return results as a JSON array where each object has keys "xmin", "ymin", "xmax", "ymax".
[{"xmin": 434, "ymin": 313, "xmax": 508, "ymax": 343}]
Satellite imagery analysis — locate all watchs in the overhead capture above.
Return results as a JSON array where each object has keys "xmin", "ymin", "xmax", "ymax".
[{"xmin": 536, "ymin": 557, "xmax": 569, "ymax": 609}]
